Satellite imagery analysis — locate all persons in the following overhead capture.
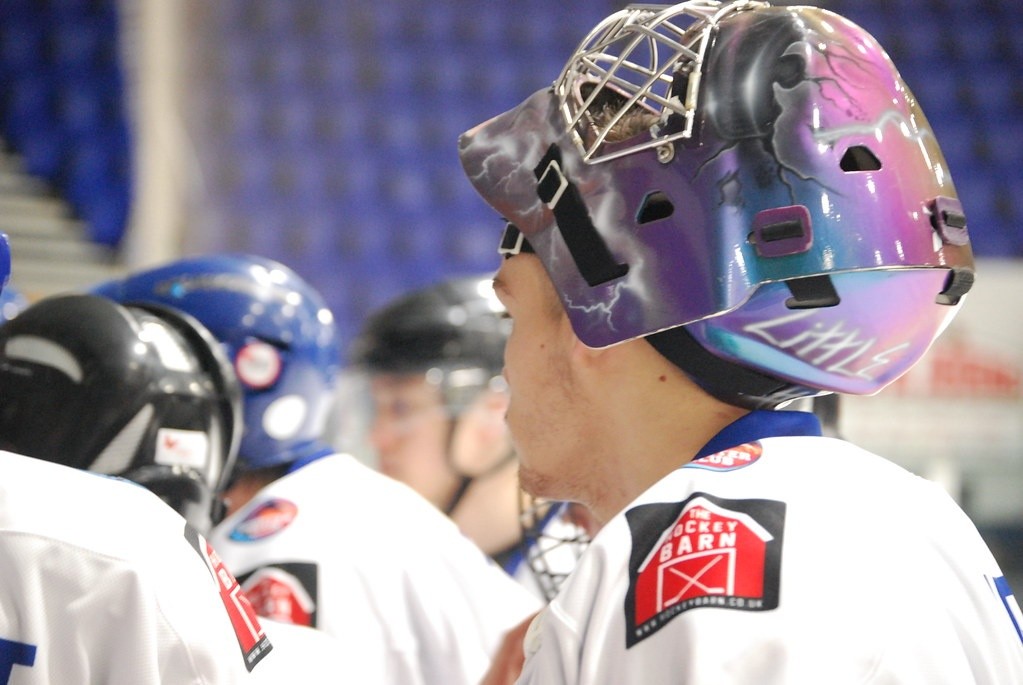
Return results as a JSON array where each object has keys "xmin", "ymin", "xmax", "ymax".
[
  {"xmin": 460, "ymin": 0, "xmax": 1023, "ymax": 684},
  {"xmin": 347, "ymin": 273, "xmax": 589, "ymax": 598},
  {"xmin": 83, "ymin": 256, "xmax": 541, "ymax": 685},
  {"xmin": 1, "ymin": 235, "xmax": 376, "ymax": 685}
]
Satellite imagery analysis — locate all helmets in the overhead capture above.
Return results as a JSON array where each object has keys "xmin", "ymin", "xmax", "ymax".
[
  {"xmin": 339, "ymin": 274, "xmax": 513, "ymax": 478},
  {"xmin": 457, "ymin": 0, "xmax": 977, "ymax": 411},
  {"xmin": 91, "ymin": 257, "xmax": 334, "ymax": 477},
  {"xmin": 0, "ymin": 293, "xmax": 246, "ymax": 533}
]
